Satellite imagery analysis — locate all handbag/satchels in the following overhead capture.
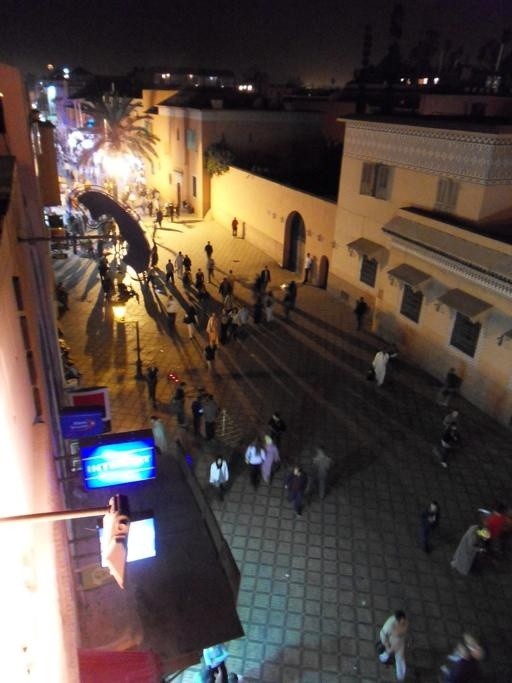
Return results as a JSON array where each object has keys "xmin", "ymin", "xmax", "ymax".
[
  {"xmin": 184, "ymin": 316, "xmax": 192, "ymax": 323},
  {"xmin": 365, "ymin": 366, "xmax": 375, "ymax": 382}
]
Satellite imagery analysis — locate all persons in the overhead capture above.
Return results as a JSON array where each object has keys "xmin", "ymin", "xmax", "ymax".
[
  {"xmin": 440, "ymin": 627, "xmax": 489, "ymax": 683},
  {"xmin": 372, "ymin": 343, "xmax": 399, "ymax": 386},
  {"xmin": 202, "ymin": 644, "xmax": 238, "ymax": 683},
  {"xmin": 421, "ymin": 500, "xmax": 512, "ymax": 576},
  {"xmin": 147, "ymin": 362, "xmax": 333, "ymax": 515},
  {"xmin": 378, "ymin": 610, "xmax": 412, "ymax": 683},
  {"xmin": 432, "ymin": 367, "xmax": 466, "ymax": 468},
  {"xmin": 303, "ymin": 253, "xmax": 312, "ymax": 282},
  {"xmin": 64, "ymin": 213, "xmax": 88, "ymax": 249},
  {"xmin": 148, "ymin": 198, "xmax": 180, "ymax": 228},
  {"xmin": 232, "ymin": 217, "xmax": 239, "ymax": 236},
  {"xmin": 98, "ymin": 241, "xmax": 297, "ymax": 376},
  {"xmin": 353, "ymin": 296, "xmax": 368, "ymax": 331},
  {"xmin": 56, "ymin": 282, "xmax": 69, "ymax": 313}
]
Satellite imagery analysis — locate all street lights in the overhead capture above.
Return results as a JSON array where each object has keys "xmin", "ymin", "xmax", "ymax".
[{"xmin": 112, "ymin": 305, "xmax": 144, "ymax": 380}]
